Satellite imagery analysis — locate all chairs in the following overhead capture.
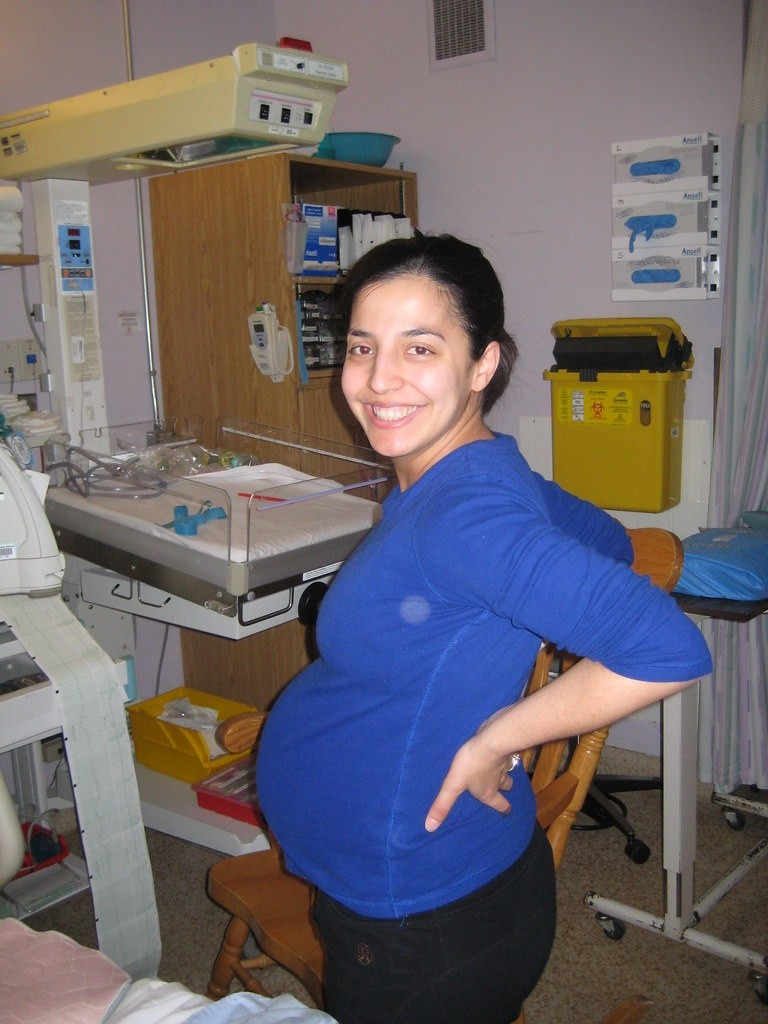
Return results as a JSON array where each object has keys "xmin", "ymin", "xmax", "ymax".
[{"xmin": 203, "ymin": 528, "xmax": 685, "ymax": 1024}]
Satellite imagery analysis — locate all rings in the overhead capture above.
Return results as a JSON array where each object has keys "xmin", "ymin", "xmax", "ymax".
[{"xmin": 507, "ymin": 754, "xmax": 520, "ymax": 771}]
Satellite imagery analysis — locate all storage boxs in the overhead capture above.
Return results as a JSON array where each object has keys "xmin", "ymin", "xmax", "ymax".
[{"xmin": 125, "ymin": 687, "xmax": 268, "ymax": 830}]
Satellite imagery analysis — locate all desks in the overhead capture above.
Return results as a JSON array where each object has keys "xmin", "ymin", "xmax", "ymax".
[{"xmin": 584, "ymin": 590, "xmax": 767, "ymax": 1006}]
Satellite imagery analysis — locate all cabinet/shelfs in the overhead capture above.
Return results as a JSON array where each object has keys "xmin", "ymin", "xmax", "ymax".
[{"xmin": 148, "ymin": 152, "xmax": 419, "ymax": 503}]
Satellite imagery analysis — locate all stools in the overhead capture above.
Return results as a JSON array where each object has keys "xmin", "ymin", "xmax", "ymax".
[{"xmin": 533, "ymin": 651, "xmax": 666, "ymax": 864}]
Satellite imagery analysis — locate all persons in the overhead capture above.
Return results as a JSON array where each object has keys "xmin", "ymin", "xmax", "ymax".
[{"xmin": 254, "ymin": 233, "xmax": 712, "ymax": 1024}]
